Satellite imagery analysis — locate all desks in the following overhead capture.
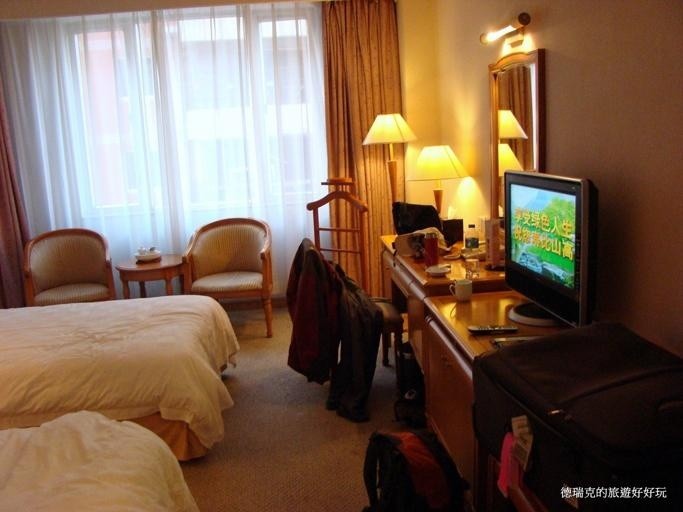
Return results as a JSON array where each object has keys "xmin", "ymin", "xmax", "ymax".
[
  {"xmin": 422, "ymin": 290, "xmax": 578, "ymax": 512},
  {"xmin": 381, "ymin": 235, "xmax": 512, "ymax": 374}
]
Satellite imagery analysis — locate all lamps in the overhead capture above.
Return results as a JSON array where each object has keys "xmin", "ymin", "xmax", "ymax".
[
  {"xmin": 479, "ymin": 12, "xmax": 531, "ymax": 45},
  {"xmin": 362, "ymin": 112, "xmax": 418, "ymax": 236},
  {"xmin": 498, "ymin": 109, "xmax": 528, "ymax": 177},
  {"xmin": 407, "ymin": 145, "xmax": 468, "ymax": 217}
]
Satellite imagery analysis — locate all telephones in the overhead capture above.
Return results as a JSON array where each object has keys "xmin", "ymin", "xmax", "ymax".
[{"xmin": 461, "ymin": 244, "xmax": 506, "ymax": 263}]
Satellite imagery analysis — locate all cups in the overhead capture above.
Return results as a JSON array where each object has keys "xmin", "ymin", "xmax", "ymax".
[
  {"xmin": 449, "ymin": 279, "xmax": 473, "ymax": 302},
  {"xmin": 138, "ymin": 246, "xmax": 154, "ymax": 256}
]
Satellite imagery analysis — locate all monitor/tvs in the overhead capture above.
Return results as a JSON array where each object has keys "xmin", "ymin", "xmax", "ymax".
[{"xmin": 503, "ymin": 169, "xmax": 600, "ymax": 331}]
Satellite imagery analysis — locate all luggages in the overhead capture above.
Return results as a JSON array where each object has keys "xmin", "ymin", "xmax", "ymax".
[{"xmin": 473, "ymin": 321, "xmax": 682, "ymax": 511}]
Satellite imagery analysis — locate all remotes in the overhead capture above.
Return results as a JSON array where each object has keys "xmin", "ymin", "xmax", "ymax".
[{"xmin": 465, "ymin": 324, "xmax": 519, "ymax": 335}]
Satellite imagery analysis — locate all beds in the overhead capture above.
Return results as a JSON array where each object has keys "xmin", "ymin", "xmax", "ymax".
[
  {"xmin": 0, "ymin": 295, "xmax": 239, "ymax": 462},
  {"xmin": 0, "ymin": 410, "xmax": 200, "ymax": 512}
]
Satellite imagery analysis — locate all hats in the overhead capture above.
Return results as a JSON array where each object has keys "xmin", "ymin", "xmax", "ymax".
[{"xmin": 395, "ymin": 227, "xmax": 447, "ymax": 258}]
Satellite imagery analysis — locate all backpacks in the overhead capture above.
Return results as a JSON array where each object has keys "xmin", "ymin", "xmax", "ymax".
[{"xmin": 363, "ymin": 429, "xmax": 465, "ymax": 512}]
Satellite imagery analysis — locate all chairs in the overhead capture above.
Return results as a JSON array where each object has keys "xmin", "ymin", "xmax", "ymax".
[
  {"xmin": 182, "ymin": 216, "xmax": 272, "ymax": 338},
  {"xmin": 302, "ymin": 239, "xmax": 404, "ymax": 395},
  {"xmin": 364, "ymin": 433, "xmax": 469, "ymax": 512},
  {"xmin": 22, "ymin": 228, "xmax": 118, "ymax": 307}
]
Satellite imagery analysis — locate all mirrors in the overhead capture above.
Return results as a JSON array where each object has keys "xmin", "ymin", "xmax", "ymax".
[{"xmin": 487, "ymin": 47, "xmax": 546, "ymax": 246}]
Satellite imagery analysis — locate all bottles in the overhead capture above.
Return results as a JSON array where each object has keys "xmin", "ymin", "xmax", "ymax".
[{"xmin": 424, "ymin": 232, "xmax": 439, "ymax": 267}]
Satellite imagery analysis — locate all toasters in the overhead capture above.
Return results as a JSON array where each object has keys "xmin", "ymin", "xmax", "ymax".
[{"xmin": 464, "ymin": 224, "xmax": 480, "ymax": 278}]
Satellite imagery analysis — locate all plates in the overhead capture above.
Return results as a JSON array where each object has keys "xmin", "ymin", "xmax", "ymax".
[
  {"xmin": 425, "ymin": 267, "xmax": 451, "ymax": 278},
  {"xmin": 134, "ymin": 252, "xmax": 160, "ymax": 261}
]
